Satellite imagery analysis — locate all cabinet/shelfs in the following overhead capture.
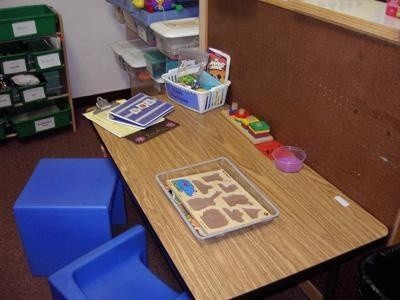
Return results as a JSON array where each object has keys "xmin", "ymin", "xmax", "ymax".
[{"xmin": 0, "ymin": 5, "xmax": 77, "ymax": 139}]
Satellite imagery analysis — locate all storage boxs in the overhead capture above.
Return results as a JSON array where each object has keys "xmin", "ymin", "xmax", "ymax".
[{"xmin": 107, "ymin": 0, "xmax": 200, "ymax": 99}]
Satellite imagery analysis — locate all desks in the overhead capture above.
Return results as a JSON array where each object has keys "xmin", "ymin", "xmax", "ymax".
[{"xmin": 86, "ymin": 94, "xmax": 390, "ymax": 299}]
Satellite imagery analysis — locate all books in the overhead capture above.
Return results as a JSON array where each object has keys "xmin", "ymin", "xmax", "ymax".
[{"xmin": 207, "ymin": 46, "xmax": 232, "ymax": 106}]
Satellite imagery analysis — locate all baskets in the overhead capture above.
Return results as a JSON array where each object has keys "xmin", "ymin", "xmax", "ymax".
[{"xmin": 161, "ymin": 67, "xmax": 232, "ymax": 114}]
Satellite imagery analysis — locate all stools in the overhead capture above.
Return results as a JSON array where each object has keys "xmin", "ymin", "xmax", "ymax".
[{"xmin": 9, "ymin": 157, "xmax": 188, "ymax": 300}]
